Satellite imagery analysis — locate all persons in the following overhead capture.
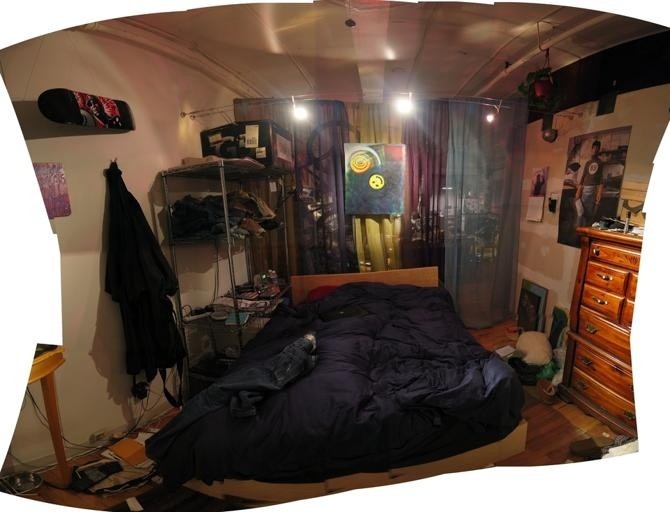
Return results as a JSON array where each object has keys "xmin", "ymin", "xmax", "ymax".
[
  {"xmin": 575, "ymin": 141, "xmax": 608, "ymax": 226},
  {"xmin": 85, "ymin": 95, "xmax": 104, "ymax": 118}
]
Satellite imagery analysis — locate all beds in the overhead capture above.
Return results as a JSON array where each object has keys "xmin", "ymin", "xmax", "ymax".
[{"xmin": 144, "ymin": 264, "xmax": 525, "ymax": 506}]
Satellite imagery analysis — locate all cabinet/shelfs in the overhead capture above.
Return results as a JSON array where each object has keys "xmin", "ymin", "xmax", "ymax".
[
  {"xmin": 160, "ymin": 162, "xmax": 289, "ymax": 392},
  {"xmin": 561, "ymin": 222, "xmax": 644, "ymax": 443}
]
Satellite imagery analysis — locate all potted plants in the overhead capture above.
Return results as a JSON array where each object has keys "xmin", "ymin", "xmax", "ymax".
[{"xmin": 518, "ymin": 49, "xmax": 563, "ymax": 107}]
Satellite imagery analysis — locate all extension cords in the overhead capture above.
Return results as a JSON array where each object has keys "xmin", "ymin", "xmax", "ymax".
[{"xmin": 181, "ymin": 307, "xmax": 213, "ymax": 323}]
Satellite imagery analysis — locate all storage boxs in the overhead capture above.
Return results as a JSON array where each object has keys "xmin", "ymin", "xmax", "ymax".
[{"xmin": 200, "ymin": 117, "xmax": 296, "ymax": 167}]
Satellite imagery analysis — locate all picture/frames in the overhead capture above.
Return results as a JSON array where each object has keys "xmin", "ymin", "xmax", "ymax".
[{"xmin": 517, "ymin": 279, "xmax": 550, "ymax": 332}]
